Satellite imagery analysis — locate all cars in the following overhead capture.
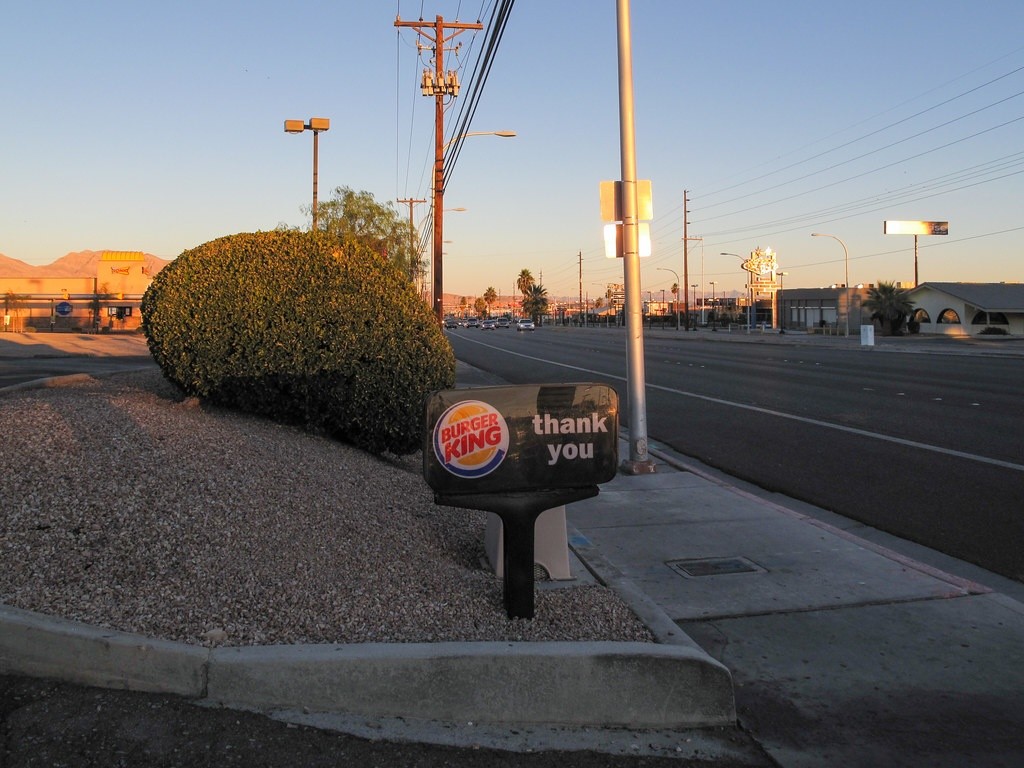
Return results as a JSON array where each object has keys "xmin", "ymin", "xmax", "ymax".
[
  {"xmin": 516, "ymin": 318, "xmax": 535, "ymax": 331},
  {"xmin": 443, "ymin": 318, "xmax": 483, "ymax": 329},
  {"xmin": 480, "ymin": 320, "xmax": 495, "ymax": 331}
]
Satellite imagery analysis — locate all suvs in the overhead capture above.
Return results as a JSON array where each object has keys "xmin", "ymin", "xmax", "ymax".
[{"xmin": 495, "ymin": 317, "xmax": 510, "ymax": 328}]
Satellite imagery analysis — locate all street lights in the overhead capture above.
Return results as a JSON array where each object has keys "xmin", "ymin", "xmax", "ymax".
[
  {"xmin": 811, "ymin": 233, "xmax": 849, "ymax": 339},
  {"xmin": 429, "ymin": 130, "xmax": 517, "ymax": 310},
  {"xmin": 591, "ymin": 283, "xmax": 610, "ymax": 327},
  {"xmin": 646, "ymin": 292, "xmax": 652, "ymax": 330},
  {"xmin": 416, "ymin": 207, "xmax": 467, "ymax": 296},
  {"xmin": 720, "ymin": 253, "xmax": 751, "ymax": 335},
  {"xmin": 691, "ymin": 284, "xmax": 699, "ymax": 330},
  {"xmin": 660, "ymin": 289, "xmax": 665, "ymax": 330},
  {"xmin": 656, "ymin": 267, "xmax": 681, "ymax": 331},
  {"xmin": 709, "ymin": 281, "xmax": 718, "ymax": 331},
  {"xmin": 283, "ymin": 117, "xmax": 330, "ymax": 234},
  {"xmin": 775, "ymin": 272, "xmax": 788, "ymax": 335}
]
